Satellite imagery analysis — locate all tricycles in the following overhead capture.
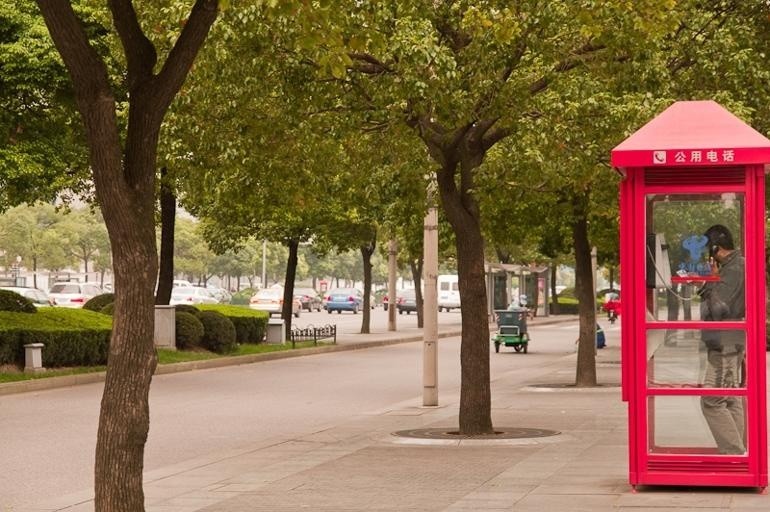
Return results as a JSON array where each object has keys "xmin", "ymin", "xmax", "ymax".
[{"xmin": 492, "ymin": 304, "xmax": 528, "ymax": 354}]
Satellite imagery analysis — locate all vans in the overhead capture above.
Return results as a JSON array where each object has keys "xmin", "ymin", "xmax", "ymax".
[{"xmin": 438, "ymin": 275, "xmax": 462, "ymax": 312}]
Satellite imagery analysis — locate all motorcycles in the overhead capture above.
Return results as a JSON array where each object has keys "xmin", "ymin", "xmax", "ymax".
[{"xmin": 606, "ymin": 300, "xmax": 618, "ymax": 322}]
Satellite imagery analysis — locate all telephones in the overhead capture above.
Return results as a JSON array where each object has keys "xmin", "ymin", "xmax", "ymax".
[{"xmin": 708, "ymin": 244, "xmax": 719, "ymax": 262}]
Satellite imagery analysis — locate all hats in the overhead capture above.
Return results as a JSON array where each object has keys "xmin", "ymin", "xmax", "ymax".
[{"xmin": 520, "ymin": 295, "xmax": 528, "ymax": 305}]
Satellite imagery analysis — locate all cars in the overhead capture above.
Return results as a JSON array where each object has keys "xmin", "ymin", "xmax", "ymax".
[
  {"xmin": 1, "ymin": 285, "xmax": 54, "ymax": 307},
  {"xmin": 329, "ymin": 288, "xmax": 364, "ymax": 314},
  {"xmin": 102, "ymin": 272, "xmax": 233, "ymax": 306},
  {"xmin": 382, "ymin": 289, "xmax": 420, "ymax": 315},
  {"xmin": 247, "ymin": 285, "xmax": 302, "ymax": 317},
  {"xmin": 48, "ymin": 281, "xmax": 104, "ymax": 308},
  {"xmin": 295, "ymin": 290, "xmax": 323, "ymax": 311}
]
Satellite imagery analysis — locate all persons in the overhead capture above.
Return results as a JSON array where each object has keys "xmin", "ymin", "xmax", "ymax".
[
  {"xmin": 605, "ymin": 289, "xmax": 619, "ymax": 321},
  {"xmin": 508, "ymin": 295, "xmax": 536, "ymax": 320},
  {"xmin": 575, "ymin": 323, "xmax": 605, "ymax": 348},
  {"xmin": 688, "ymin": 224, "xmax": 749, "ymax": 455}
]
